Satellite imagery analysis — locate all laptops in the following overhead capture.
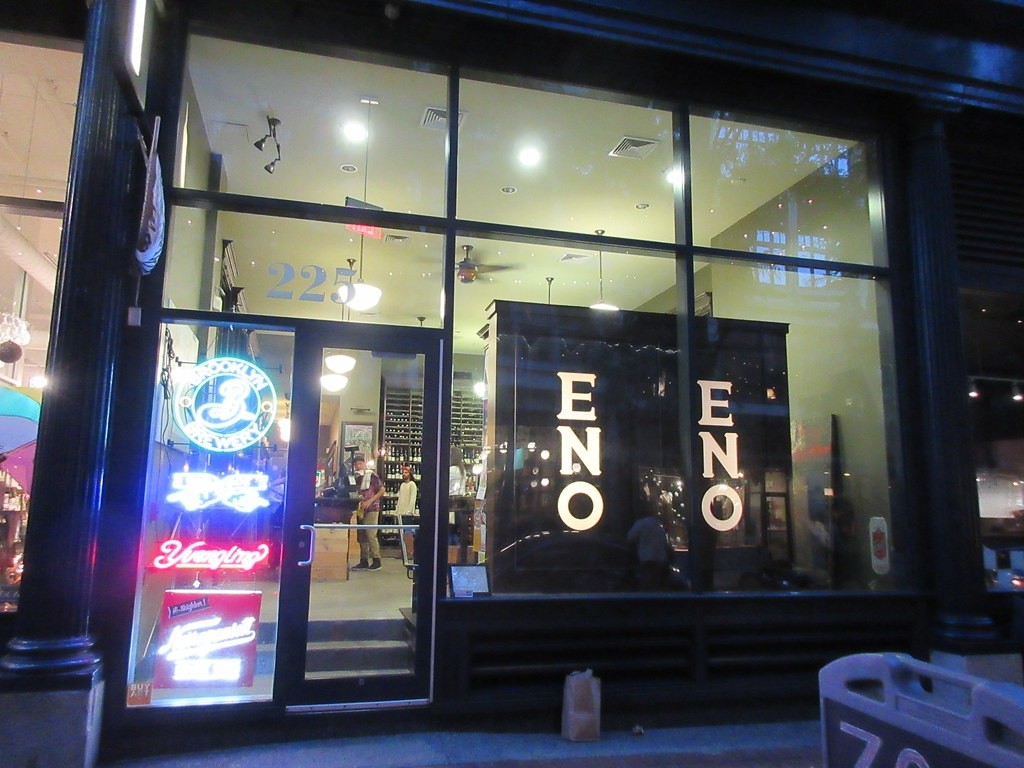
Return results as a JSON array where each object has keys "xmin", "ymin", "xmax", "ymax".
[{"xmin": 448, "ymin": 562, "xmax": 493, "ymax": 598}]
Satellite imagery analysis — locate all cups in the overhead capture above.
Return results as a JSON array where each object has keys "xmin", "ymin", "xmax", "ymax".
[{"xmin": 349, "ymin": 492, "xmax": 358, "ymax": 499}]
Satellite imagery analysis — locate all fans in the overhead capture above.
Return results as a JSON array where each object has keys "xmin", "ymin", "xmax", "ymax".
[{"xmin": 415, "ymin": 243, "xmax": 521, "ymax": 284}]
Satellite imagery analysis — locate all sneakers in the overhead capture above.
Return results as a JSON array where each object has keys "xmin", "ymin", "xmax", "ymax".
[
  {"xmin": 367, "ymin": 558, "xmax": 381, "ymax": 571},
  {"xmin": 352, "ymin": 559, "xmax": 368, "ymax": 571}
]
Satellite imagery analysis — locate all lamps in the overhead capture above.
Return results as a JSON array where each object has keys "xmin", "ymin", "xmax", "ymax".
[
  {"xmin": 260, "ymin": 444, "xmax": 278, "ymax": 452},
  {"xmin": 276, "ymin": 393, "xmax": 290, "ymax": 442},
  {"xmin": 264, "ymin": 118, "xmax": 283, "ymax": 174},
  {"xmin": 339, "ymin": 93, "xmax": 383, "ymax": 312},
  {"xmin": 0, "ymin": 313, "xmax": 30, "ymax": 369},
  {"xmin": 325, "ymin": 259, "xmax": 357, "ymax": 374},
  {"xmin": 321, "ymin": 373, "xmax": 350, "ymax": 392},
  {"xmin": 264, "ymin": 364, "xmax": 284, "ymax": 374},
  {"xmin": 253, "ymin": 115, "xmax": 272, "ymax": 151},
  {"xmin": 458, "ymin": 269, "xmax": 478, "ymax": 284},
  {"xmin": 168, "ymin": 438, "xmax": 198, "ymax": 455}
]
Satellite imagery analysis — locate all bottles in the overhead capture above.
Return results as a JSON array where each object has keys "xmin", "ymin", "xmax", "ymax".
[
  {"xmin": 358, "ymin": 499, "xmax": 365, "ymax": 519},
  {"xmin": 382, "ymin": 411, "xmax": 483, "ymax": 545}
]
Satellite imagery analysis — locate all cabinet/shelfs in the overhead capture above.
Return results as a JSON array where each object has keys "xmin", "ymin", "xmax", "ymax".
[{"xmin": 375, "ymin": 385, "xmax": 486, "ymax": 546}]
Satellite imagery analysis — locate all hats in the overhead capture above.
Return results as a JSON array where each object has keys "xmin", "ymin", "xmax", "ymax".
[{"xmin": 401, "ymin": 465, "xmax": 414, "ymax": 475}]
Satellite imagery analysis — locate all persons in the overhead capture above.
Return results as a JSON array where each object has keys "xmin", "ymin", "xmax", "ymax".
[
  {"xmin": 449, "ymin": 445, "xmax": 466, "ymax": 496},
  {"xmin": 396, "ymin": 465, "xmax": 417, "ymax": 526},
  {"xmin": 352, "ymin": 455, "xmax": 386, "ymax": 572},
  {"xmin": 624, "ymin": 501, "xmax": 671, "ymax": 593}
]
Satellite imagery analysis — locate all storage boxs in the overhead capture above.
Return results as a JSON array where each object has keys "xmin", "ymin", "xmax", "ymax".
[{"xmin": 448, "ymin": 543, "xmax": 463, "ymax": 563}]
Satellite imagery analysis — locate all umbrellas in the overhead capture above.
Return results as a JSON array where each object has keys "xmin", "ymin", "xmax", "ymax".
[{"xmin": 0, "ymin": 386, "xmax": 42, "ymax": 496}]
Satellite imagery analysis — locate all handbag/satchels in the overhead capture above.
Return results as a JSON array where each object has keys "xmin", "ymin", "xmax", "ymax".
[{"xmin": 560, "ymin": 669, "xmax": 602, "ymax": 742}]
